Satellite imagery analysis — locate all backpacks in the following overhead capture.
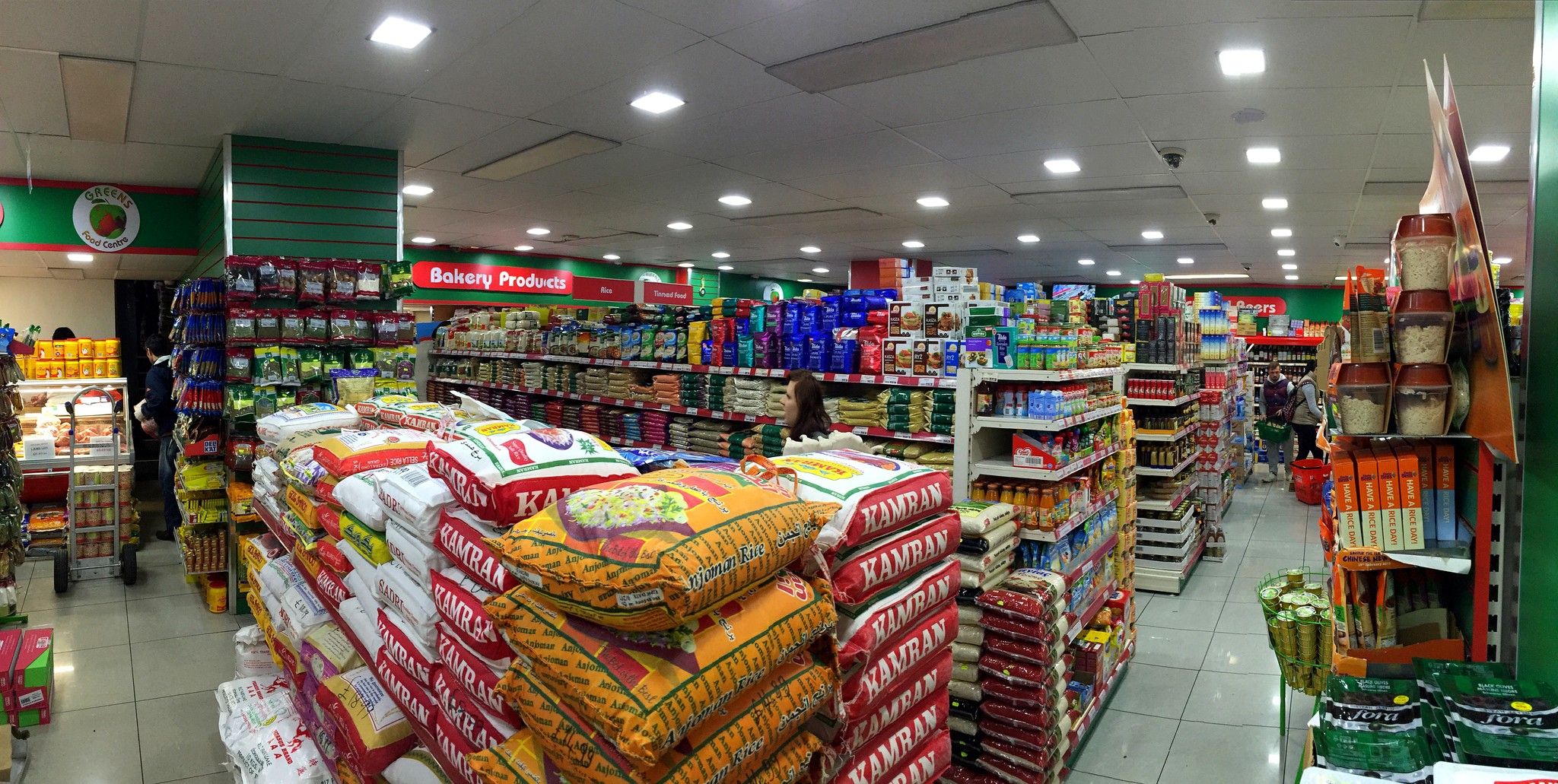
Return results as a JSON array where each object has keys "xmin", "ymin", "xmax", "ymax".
[{"xmin": 1281, "ymin": 380, "xmax": 1316, "ymax": 423}]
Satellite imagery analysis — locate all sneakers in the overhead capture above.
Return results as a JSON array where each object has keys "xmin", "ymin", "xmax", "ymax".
[
  {"xmin": 1262, "ymin": 471, "xmax": 1278, "ymax": 482},
  {"xmin": 1285, "ymin": 471, "xmax": 1292, "ymax": 480}
]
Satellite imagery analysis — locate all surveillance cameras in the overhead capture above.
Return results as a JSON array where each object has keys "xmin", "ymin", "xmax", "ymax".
[
  {"xmin": 1241, "ymin": 263, "xmax": 1252, "ymax": 271},
  {"xmin": 1334, "ymin": 237, "xmax": 1345, "ymax": 248},
  {"xmin": 1205, "ymin": 214, "xmax": 1219, "ymax": 225},
  {"xmin": 1322, "ymin": 282, "xmax": 1331, "ymax": 290},
  {"xmin": 1159, "ymin": 147, "xmax": 1187, "ymax": 171}
]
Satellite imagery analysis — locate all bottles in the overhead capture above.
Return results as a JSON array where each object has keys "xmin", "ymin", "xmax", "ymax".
[
  {"xmin": 1254, "ymin": 408, "xmax": 1262, "ymax": 421},
  {"xmin": 972, "ymin": 476, "xmax": 1069, "ymax": 531},
  {"xmin": 977, "ymin": 379, "xmax": 993, "ymax": 417},
  {"xmin": 995, "ymin": 383, "xmax": 1089, "ymax": 420},
  {"xmin": 1234, "ymin": 397, "xmax": 1246, "ymax": 416},
  {"xmin": 1254, "ymin": 389, "xmax": 1259, "ymax": 403},
  {"xmin": 1246, "ymin": 366, "xmax": 1305, "ymax": 384},
  {"xmin": 1318, "ymin": 391, "xmax": 1323, "ymax": 406},
  {"xmin": 1246, "ymin": 344, "xmax": 1318, "ymax": 363},
  {"xmin": 1206, "ymin": 525, "xmax": 1226, "ymax": 543},
  {"xmin": 1134, "ymin": 413, "xmax": 1184, "ymax": 430},
  {"xmin": 1141, "ymin": 431, "xmax": 1194, "ymax": 467}
]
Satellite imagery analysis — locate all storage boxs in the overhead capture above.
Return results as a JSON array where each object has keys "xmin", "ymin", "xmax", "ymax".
[
  {"xmin": 0, "ymin": 628, "xmax": 54, "ymax": 728},
  {"xmin": 885, "ymin": 266, "xmax": 1258, "ymax": 718},
  {"xmin": 1327, "ymin": 438, "xmax": 1456, "ymax": 552}
]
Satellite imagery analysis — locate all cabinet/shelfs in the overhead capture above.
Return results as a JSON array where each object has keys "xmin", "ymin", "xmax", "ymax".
[
  {"xmin": 1126, "ymin": 360, "xmax": 1211, "ymax": 595},
  {"xmin": 0, "ymin": 374, "xmax": 136, "ymax": 563},
  {"xmin": 1238, "ymin": 334, "xmax": 1326, "ymax": 463},
  {"xmin": 1202, "ymin": 358, "xmax": 1249, "ymax": 520},
  {"xmin": 425, "ymin": 346, "xmax": 1133, "ymax": 784},
  {"xmin": 170, "ymin": 430, "xmax": 256, "ymax": 615},
  {"xmin": 1306, "ymin": 430, "xmax": 1497, "ymax": 672}
]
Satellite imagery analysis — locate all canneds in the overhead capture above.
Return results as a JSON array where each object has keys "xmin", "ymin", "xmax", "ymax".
[
  {"xmin": 13, "ymin": 337, "xmax": 122, "ymax": 379},
  {"xmin": 1136, "ymin": 496, "xmax": 1206, "ymax": 563},
  {"xmin": 1206, "ymin": 547, "xmax": 1222, "ymax": 557},
  {"xmin": 1017, "ymin": 322, "xmax": 1121, "ymax": 371},
  {"xmin": 1075, "ymin": 376, "xmax": 1120, "ymax": 411}
]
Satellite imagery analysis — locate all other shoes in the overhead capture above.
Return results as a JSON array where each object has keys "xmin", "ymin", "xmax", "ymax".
[
  {"xmin": 155, "ymin": 530, "xmax": 176, "ymax": 542},
  {"xmin": 1289, "ymin": 479, "xmax": 1296, "ymax": 492}
]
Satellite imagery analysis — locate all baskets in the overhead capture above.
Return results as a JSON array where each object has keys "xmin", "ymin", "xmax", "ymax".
[
  {"xmin": 1256, "ymin": 413, "xmax": 1292, "ymax": 443},
  {"xmin": 1289, "ymin": 460, "xmax": 1332, "ymax": 505}
]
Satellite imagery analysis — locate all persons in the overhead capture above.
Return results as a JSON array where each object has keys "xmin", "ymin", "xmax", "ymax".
[
  {"xmin": 52, "ymin": 327, "xmax": 76, "ymax": 340},
  {"xmin": 1259, "ymin": 361, "xmax": 1296, "ymax": 483},
  {"xmin": 1288, "ymin": 359, "xmax": 1322, "ymax": 493},
  {"xmin": 134, "ymin": 333, "xmax": 183, "ymax": 541},
  {"xmin": 779, "ymin": 369, "xmax": 832, "ymax": 443}
]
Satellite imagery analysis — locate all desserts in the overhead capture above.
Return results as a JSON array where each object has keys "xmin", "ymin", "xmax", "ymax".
[{"xmin": 898, "ymin": 311, "xmax": 960, "ymax": 368}]
